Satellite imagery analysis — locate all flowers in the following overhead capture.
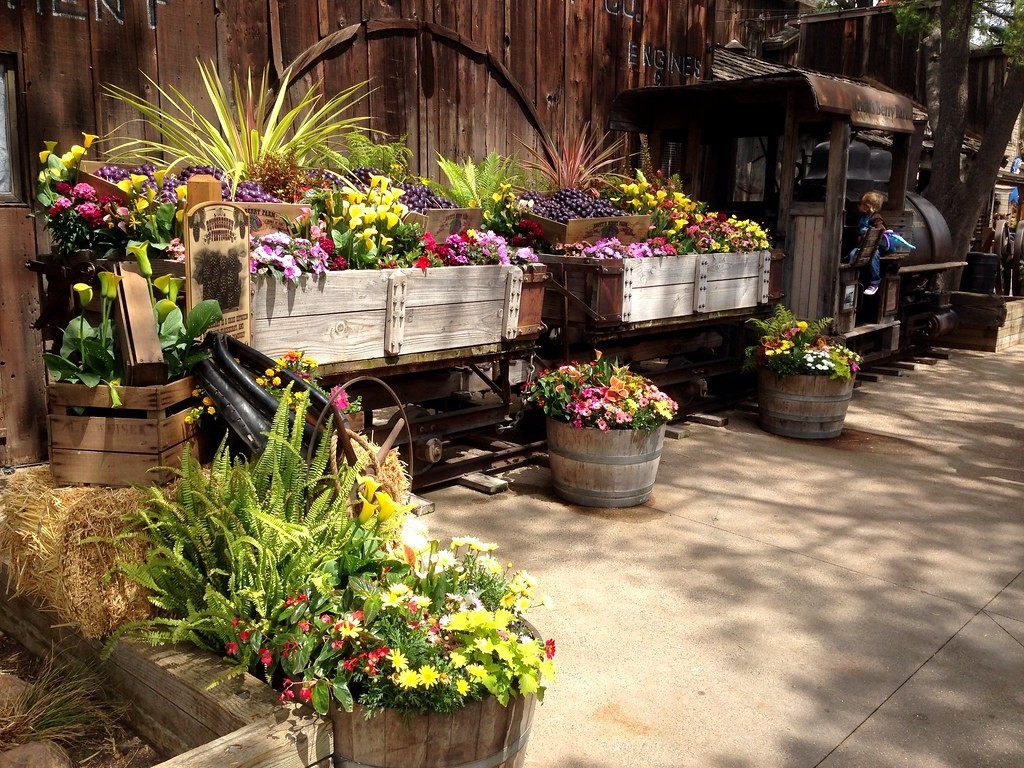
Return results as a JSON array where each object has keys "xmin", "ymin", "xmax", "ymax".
[
  {"xmin": 24, "ymin": 132, "xmax": 99, "ymax": 220},
  {"xmin": 374, "ymin": 222, "xmax": 443, "ymax": 269},
  {"xmin": 519, "ymin": 349, "xmax": 679, "ymax": 440},
  {"xmin": 42, "ymin": 238, "xmax": 224, "ymax": 416},
  {"xmin": 428, "ymin": 118, "xmax": 775, "ymax": 259},
  {"xmin": 43, "ymin": 183, "xmax": 122, "ymax": 260},
  {"xmin": 184, "ymin": 347, "xmax": 363, "ymax": 430},
  {"xmin": 94, "ymin": 169, "xmax": 187, "ymax": 260},
  {"xmin": 445, "ymin": 228, "xmax": 538, "ymax": 265},
  {"xmin": 745, "ymin": 304, "xmax": 864, "ymax": 384},
  {"xmin": 321, "ymin": 173, "xmax": 408, "ymax": 269},
  {"xmin": 225, "ymin": 475, "xmax": 557, "ymax": 737}
]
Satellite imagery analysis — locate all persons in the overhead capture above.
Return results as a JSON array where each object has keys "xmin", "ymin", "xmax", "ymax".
[{"xmin": 845, "ymin": 191, "xmax": 888, "ymax": 295}]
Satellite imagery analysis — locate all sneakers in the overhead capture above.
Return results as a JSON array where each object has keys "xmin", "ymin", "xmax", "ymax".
[{"xmin": 863, "ymin": 285, "xmax": 878, "ymax": 295}]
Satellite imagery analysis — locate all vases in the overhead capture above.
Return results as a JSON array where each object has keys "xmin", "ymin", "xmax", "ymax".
[
  {"xmin": 330, "ymin": 615, "xmax": 544, "ymax": 768},
  {"xmin": 546, "ymin": 415, "xmax": 667, "ymax": 507},
  {"xmin": 757, "ymin": 365, "xmax": 855, "ymax": 440}
]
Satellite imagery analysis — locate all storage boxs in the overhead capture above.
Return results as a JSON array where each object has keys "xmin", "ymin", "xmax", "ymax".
[{"xmin": 44, "ymin": 376, "xmax": 210, "ymax": 486}]
